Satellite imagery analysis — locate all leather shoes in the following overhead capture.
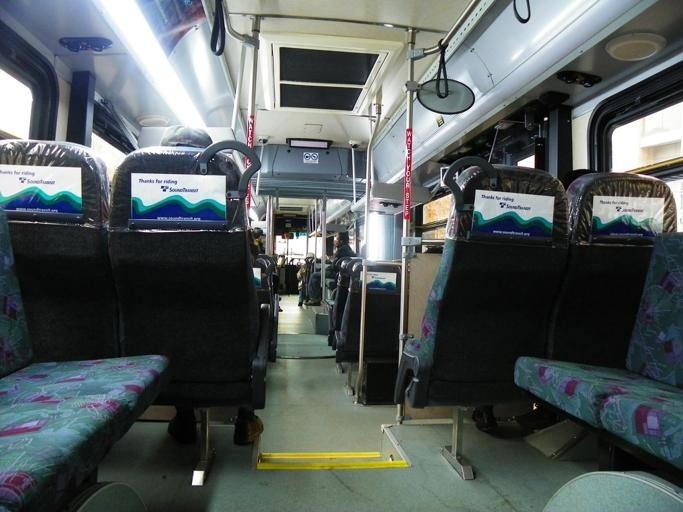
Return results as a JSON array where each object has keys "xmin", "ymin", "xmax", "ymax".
[
  {"xmin": 472, "ymin": 410, "xmax": 498, "ymax": 431},
  {"xmin": 169, "ymin": 416, "xmax": 198, "ymax": 444},
  {"xmin": 233, "ymin": 415, "xmax": 264, "ymax": 444},
  {"xmin": 304, "ymin": 300, "xmax": 321, "ymax": 306}
]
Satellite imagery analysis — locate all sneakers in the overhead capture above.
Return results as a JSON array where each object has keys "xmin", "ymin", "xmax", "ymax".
[{"xmin": 298, "ymin": 302, "xmax": 303, "ymax": 306}]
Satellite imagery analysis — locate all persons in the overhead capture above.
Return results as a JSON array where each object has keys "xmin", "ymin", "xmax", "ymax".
[
  {"xmin": 305, "ymin": 229, "xmax": 355, "ymax": 306},
  {"xmin": 254, "ymin": 227, "xmax": 266, "ymax": 255},
  {"xmin": 251, "ymin": 241, "xmax": 283, "ymax": 313},
  {"xmin": 297, "ymin": 254, "xmax": 314, "ymax": 306}
]
